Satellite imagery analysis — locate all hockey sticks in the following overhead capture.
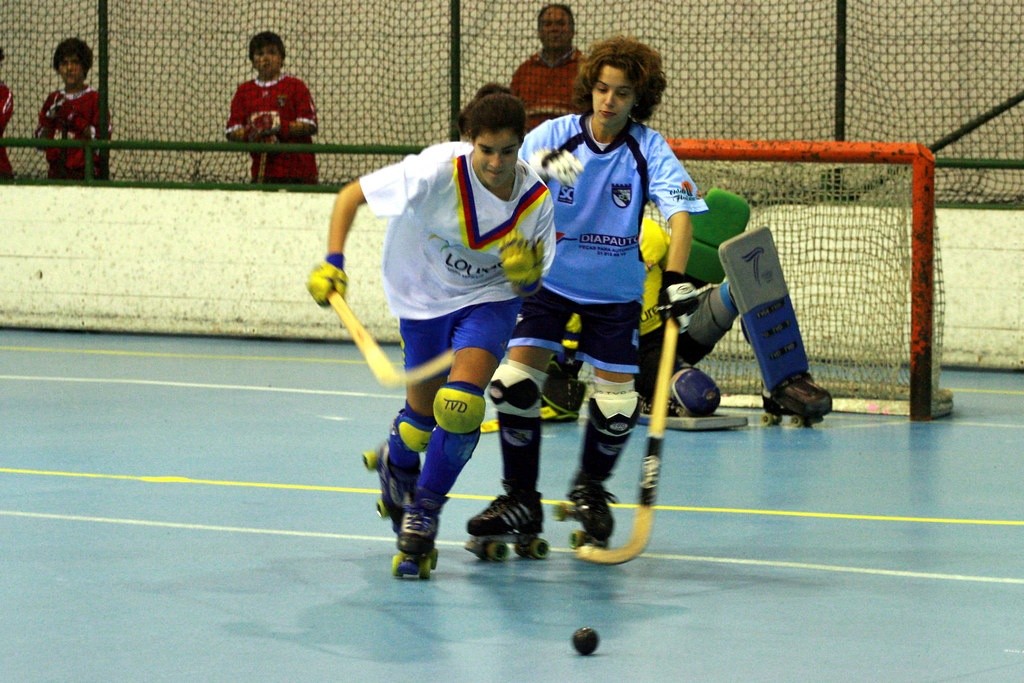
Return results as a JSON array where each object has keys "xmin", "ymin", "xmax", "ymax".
[
  {"xmin": 574, "ymin": 315, "xmax": 677, "ymax": 566},
  {"xmin": 328, "ymin": 290, "xmax": 456, "ymax": 387}
]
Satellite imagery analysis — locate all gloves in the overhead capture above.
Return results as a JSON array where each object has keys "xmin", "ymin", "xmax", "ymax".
[
  {"xmin": 527, "ymin": 147, "xmax": 585, "ymax": 186},
  {"xmin": 224, "ymin": 124, "xmax": 245, "ymax": 142},
  {"xmin": 243, "ymin": 110, "xmax": 281, "ymax": 143},
  {"xmin": 657, "ymin": 269, "xmax": 710, "ymax": 331},
  {"xmin": 496, "ymin": 228, "xmax": 545, "ymax": 293},
  {"xmin": 305, "ymin": 253, "xmax": 349, "ymax": 306}
]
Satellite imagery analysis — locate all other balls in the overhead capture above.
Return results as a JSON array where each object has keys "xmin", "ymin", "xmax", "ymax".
[{"xmin": 573, "ymin": 627, "xmax": 599, "ymax": 657}]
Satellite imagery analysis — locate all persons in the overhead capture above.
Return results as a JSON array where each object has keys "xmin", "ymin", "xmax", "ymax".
[
  {"xmin": 221, "ymin": 28, "xmax": 321, "ymax": 185},
  {"xmin": 0, "ymin": 46, "xmax": 15, "ymax": 180},
  {"xmin": 463, "ymin": 36, "xmax": 711, "ymax": 561},
  {"xmin": 507, "ymin": 5, "xmax": 589, "ymax": 138},
  {"xmin": 30, "ymin": 36, "xmax": 114, "ymax": 179},
  {"xmin": 303, "ymin": 83, "xmax": 556, "ymax": 580},
  {"xmin": 559, "ymin": 212, "xmax": 833, "ymax": 427}
]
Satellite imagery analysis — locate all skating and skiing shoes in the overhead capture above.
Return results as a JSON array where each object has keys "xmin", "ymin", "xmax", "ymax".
[
  {"xmin": 760, "ymin": 380, "xmax": 834, "ymax": 431},
  {"xmin": 553, "ymin": 472, "xmax": 617, "ymax": 547},
  {"xmin": 464, "ymin": 479, "xmax": 551, "ymax": 562},
  {"xmin": 391, "ymin": 486, "xmax": 450, "ymax": 579},
  {"xmin": 362, "ymin": 437, "xmax": 421, "ymax": 528}
]
